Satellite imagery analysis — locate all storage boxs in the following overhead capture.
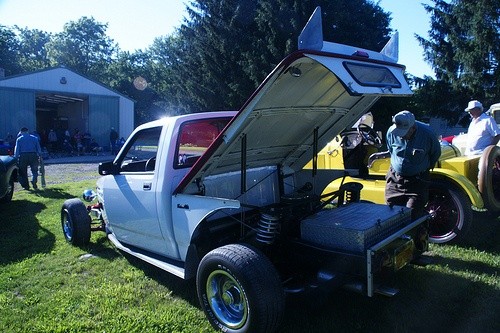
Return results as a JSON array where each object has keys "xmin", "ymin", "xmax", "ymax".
[{"xmin": 300, "ymin": 202, "xmax": 413, "ymax": 255}]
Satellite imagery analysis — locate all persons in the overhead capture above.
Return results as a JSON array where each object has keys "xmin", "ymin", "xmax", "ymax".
[
  {"xmin": 464, "ymin": 99, "xmax": 500, "ymax": 155},
  {"xmin": 13, "ymin": 127, "xmax": 42, "ymax": 190},
  {"xmin": 47, "ymin": 126, "xmax": 125, "ymax": 156},
  {"xmin": 386, "ymin": 109, "xmax": 440, "ymax": 211}
]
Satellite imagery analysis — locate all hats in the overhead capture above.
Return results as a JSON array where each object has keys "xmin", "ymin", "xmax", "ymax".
[
  {"xmin": 389, "ymin": 110, "xmax": 415, "ymax": 134},
  {"xmin": 464, "ymin": 100, "xmax": 482, "ymax": 112}
]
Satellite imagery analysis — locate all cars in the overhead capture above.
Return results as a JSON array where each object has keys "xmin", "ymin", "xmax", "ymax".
[{"xmin": 2, "ymin": 153, "xmax": 19, "ymax": 207}]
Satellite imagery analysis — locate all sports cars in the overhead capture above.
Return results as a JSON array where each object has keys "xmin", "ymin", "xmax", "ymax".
[
  {"xmin": 60, "ymin": 4, "xmax": 433, "ymax": 333},
  {"xmin": 302, "ymin": 120, "xmax": 487, "ymax": 243}
]
